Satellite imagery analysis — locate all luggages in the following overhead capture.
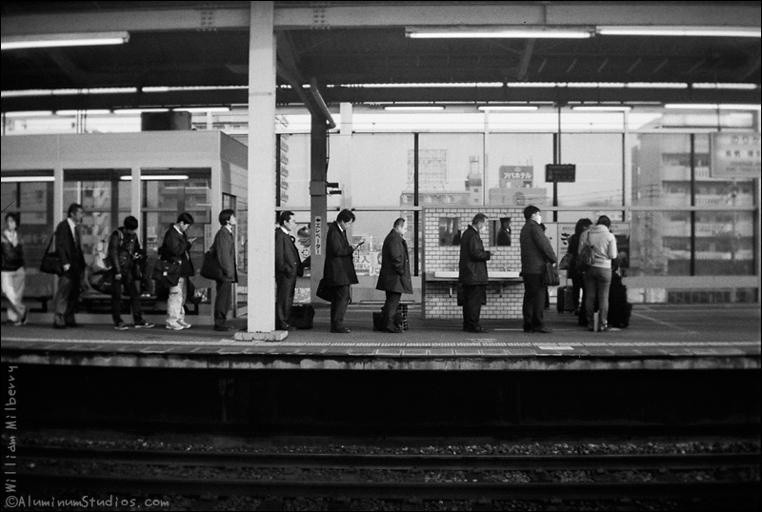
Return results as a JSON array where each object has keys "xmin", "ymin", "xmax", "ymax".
[{"xmin": 557, "ymin": 280, "xmax": 574, "ymax": 314}]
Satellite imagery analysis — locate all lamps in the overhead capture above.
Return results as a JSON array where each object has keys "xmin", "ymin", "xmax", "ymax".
[
  {"xmin": 401, "ymin": 26, "xmax": 594, "ymax": 41},
  {"xmin": 2, "ymin": 77, "xmax": 760, "ymax": 118},
  {"xmin": 595, "ymin": 22, "xmax": 759, "ymax": 43},
  {"xmin": 1, "ymin": 29, "xmax": 133, "ymax": 52}
]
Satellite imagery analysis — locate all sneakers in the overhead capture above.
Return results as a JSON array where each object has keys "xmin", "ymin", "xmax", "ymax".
[
  {"xmin": 166, "ymin": 319, "xmax": 192, "ymax": 330},
  {"xmin": 134, "ymin": 320, "xmax": 156, "ymax": 329},
  {"xmin": 113, "ymin": 322, "xmax": 129, "ymax": 330},
  {"xmin": 14, "ymin": 307, "xmax": 29, "ymax": 327}
]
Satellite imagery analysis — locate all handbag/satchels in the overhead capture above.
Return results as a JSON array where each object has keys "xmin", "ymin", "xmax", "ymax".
[
  {"xmin": 151, "ymin": 260, "xmax": 180, "ymax": 286},
  {"xmin": 289, "ymin": 304, "xmax": 315, "ymax": 329},
  {"xmin": 457, "ymin": 287, "xmax": 486, "ymax": 306},
  {"xmin": 544, "ymin": 263, "xmax": 559, "ymax": 286},
  {"xmin": 316, "ymin": 279, "xmax": 331, "ymax": 302},
  {"xmin": 41, "ymin": 252, "xmax": 63, "ymax": 277},
  {"xmin": 373, "ymin": 304, "xmax": 408, "ymax": 331},
  {"xmin": 200, "ymin": 252, "xmax": 215, "ymax": 280},
  {"xmin": 558, "ymin": 245, "xmax": 595, "ymax": 270}
]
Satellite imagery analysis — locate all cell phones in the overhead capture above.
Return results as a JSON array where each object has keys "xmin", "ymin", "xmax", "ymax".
[
  {"xmin": 192, "ymin": 237, "xmax": 197, "ymax": 242},
  {"xmin": 134, "ymin": 255, "xmax": 139, "ymax": 259},
  {"xmin": 357, "ymin": 241, "xmax": 364, "ymax": 248}
]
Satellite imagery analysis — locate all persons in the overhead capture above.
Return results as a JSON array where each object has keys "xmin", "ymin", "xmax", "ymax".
[
  {"xmin": 53, "ymin": 203, "xmax": 87, "ymax": 330},
  {"xmin": 578, "ymin": 215, "xmax": 618, "ymax": 332},
  {"xmin": 323, "ymin": 209, "xmax": 359, "ymax": 333},
  {"xmin": 107, "ymin": 216, "xmax": 156, "ymax": 331},
  {"xmin": 275, "ymin": 211, "xmax": 304, "ymax": 331},
  {"xmin": 519, "ymin": 205, "xmax": 557, "ymax": 334},
  {"xmin": 459, "ymin": 213, "xmax": 494, "ymax": 333},
  {"xmin": 211, "ymin": 209, "xmax": 238, "ymax": 332},
  {"xmin": 160, "ymin": 212, "xmax": 197, "ymax": 331},
  {"xmin": 497, "ymin": 217, "xmax": 511, "ymax": 246},
  {"xmin": 376, "ymin": 217, "xmax": 413, "ymax": 334},
  {"xmin": 1, "ymin": 213, "xmax": 29, "ymax": 326},
  {"xmin": 567, "ymin": 218, "xmax": 593, "ymax": 316}
]
